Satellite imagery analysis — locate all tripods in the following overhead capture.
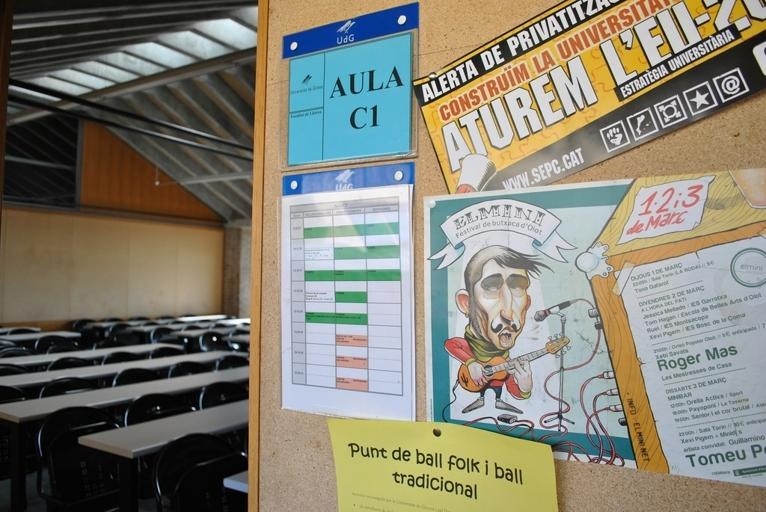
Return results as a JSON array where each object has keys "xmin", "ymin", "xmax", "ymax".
[{"xmin": 543, "ymin": 317, "xmax": 575, "ymax": 432}]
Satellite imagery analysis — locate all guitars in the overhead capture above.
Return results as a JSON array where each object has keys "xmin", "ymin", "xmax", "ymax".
[{"xmin": 458, "ymin": 332, "xmax": 571, "ymax": 393}]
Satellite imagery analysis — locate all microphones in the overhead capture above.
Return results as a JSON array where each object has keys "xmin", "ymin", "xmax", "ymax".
[{"xmin": 534, "ymin": 299, "xmax": 577, "ymax": 321}]
